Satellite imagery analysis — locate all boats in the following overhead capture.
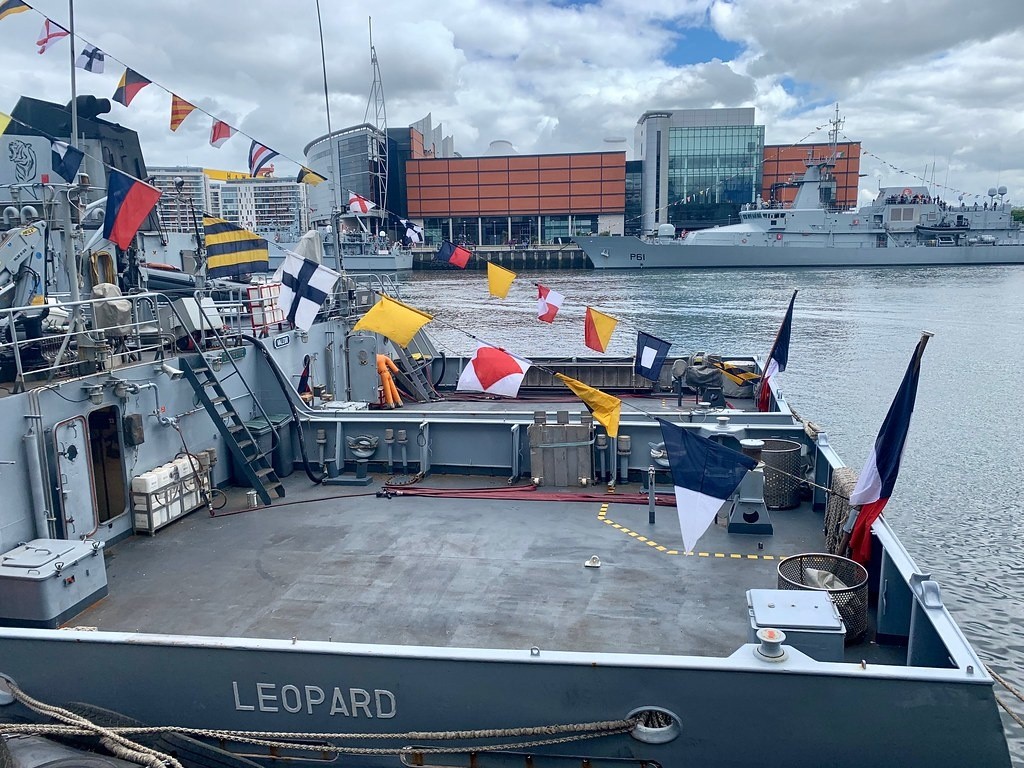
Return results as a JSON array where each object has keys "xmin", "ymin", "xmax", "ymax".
[
  {"xmin": 136, "ymin": 193, "xmax": 414, "ymax": 271},
  {"xmin": 915, "ymin": 206, "xmax": 970, "ymax": 234},
  {"xmin": 568, "ymin": 102, "xmax": 1024, "ymax": 269}
]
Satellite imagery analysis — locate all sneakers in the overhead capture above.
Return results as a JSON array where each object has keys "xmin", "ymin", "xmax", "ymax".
[{"xmin": 382, "ymin": 402, "xmax": 404, "ymax": 410}]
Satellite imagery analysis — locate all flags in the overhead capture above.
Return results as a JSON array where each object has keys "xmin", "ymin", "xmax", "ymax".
[
  {"xmin": 350, "ymin": 293, "xmax": 433, "ymax": 350},
  {"xmin": 349, "ymin": 190, "xmax": 378, "ymax": 212},
  {"xmin": 455, "ymin": 339, "xmax": 531, "ymax": 399},
  {"xmin": 488, "ymin": 262, "xmax": 516, "ymax": 299},
  {"xmin": 112, "ymin": 67, "xmax": 328, "ymax": 193},
  {"xmin": 846, "ymin": 328, "xmax": 932, "ymax": 562},
  {"xmin": 551, "ymin": 371, "xmax": 622, "ymax": 439},
  {"xmin": 75, "ymin": 44, "xmax": 107, "ymax": 73},
  {"xmin": 37, "ymin": 19, "xmax": 68, "ymax": 54},
  {"xmin": 536, "ymin": 284, "xmax": 566, "ymax": 324},
  {"xmin": 634, "ymin": 331, "xmax": 673, "ymax": 382},
  {"xmin": 203, "ymin": 214, "xmax": 270, "ymax": 282},
  {"xmin": 104, "ymin": 167, "xmax": 163, "ymax": 252},
  {"xmin": 0, "ymin": 0, "xmax": 33, "ymax": 20},
  {"xmin": 755, "ymin": 290, "xmax": 798, "ymax": 413},
  {"xmin": 398, "ymin": 215, "xmax": 426, "ymax": 245},
  {"xmin": 33, "ymin": 127, "xmax": 85, "ymax": 185},
  {"xmin": 436, "ymin": 239, "xmax": 473, "ymax": 268},
  {"xmin": 585, "ymin": 305, "xmax": 618, "ymax": 354},
  {"xmin": 279, "ymin": 249, "xmax": 341, "ymax": 332}
]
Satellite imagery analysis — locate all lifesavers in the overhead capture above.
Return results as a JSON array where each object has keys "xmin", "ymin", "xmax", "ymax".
[
  {"xmin": 777, "ymin": 233, "xmax": 783, "ymax": 240},
  {"xmin": 742, "ymin": 239, "xmax": 746, "ymax": 243}
]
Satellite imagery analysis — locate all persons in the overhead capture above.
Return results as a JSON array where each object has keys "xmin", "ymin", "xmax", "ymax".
[
  {"xmin": 886, "ymin": 193, "xmax": 939, "ymax": 204},
  {"xmin": 458, "ymin": 233, "xmax": 471, "ymax": 244},
  {"xmin": 377, "ymin": 354, "xmax": 404, "ymax": 408},
  {"xmin": 502, "ymin": 230, "xmax": 507, "ymax": 245},
  {"xmin": 962, "ymin": 201, "xmax": 997, "ymax": 211}
]
